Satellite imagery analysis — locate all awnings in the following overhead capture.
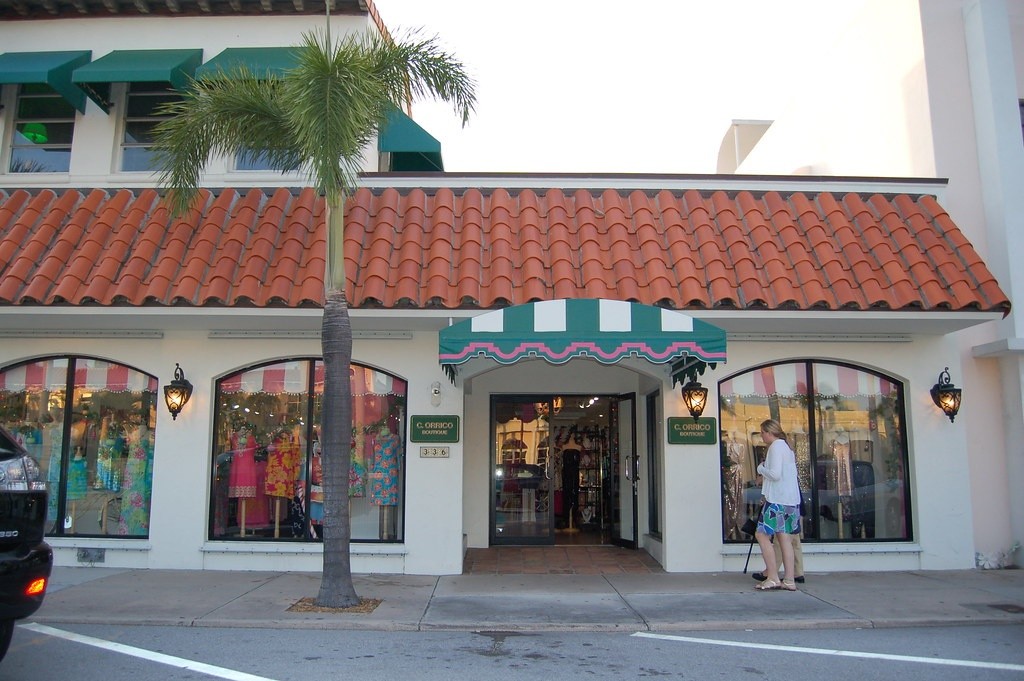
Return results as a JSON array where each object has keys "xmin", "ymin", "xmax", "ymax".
[
  {"xmin": 439, "ymin": 298, "xmax": 727, "ymax": 389},
  {"xmin": 380, "ymin": 96, "xmax": 443, "ymax": 171},
  {"xmin": 195, "ymin": 47, "xmax": 307, "ymax": 93},
  {"xmin": 72, "ymin": 48, "xmax": 203, "ymax": 115},
  {"xmin": 0, "ymin": 50, "xmax": 91, "ymax": 115}
]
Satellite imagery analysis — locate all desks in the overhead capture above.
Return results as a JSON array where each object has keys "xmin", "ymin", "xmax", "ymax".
[{"xmin": 68, "ymin": 490, "xmax": 122, "ymax": 534}]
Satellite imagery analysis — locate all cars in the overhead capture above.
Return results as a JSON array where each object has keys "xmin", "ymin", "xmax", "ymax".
[
  {"xmin": 742, "ymin": 460, "xmax": 903, "ymax": 540},
  {"xmin": 0, "ymin": 426, "xmax": 54, "ymax": 662}
]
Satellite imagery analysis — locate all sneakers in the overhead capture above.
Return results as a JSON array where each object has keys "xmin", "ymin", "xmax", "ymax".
[
  {"xmin": 780, "ymin": 575, "xmax": 805, "ymax": 584},
  {"xmin": 752, "ymin": 572, "xmax": 767, "ymax": 581}
]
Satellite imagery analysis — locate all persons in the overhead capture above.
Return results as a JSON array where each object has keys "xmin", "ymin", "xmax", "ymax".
[
  {"xmin": 227, "ymin": 426, "xmax": 401, "ymax": 505},
  {"xmin": 217, "ymin": 439, "xmax": 230, "ymax": 478},
  {"xmin": 723, "ymin": 431, "xmax": 744, "ymax": 539},
  {"xmin": 17, "ymin": 408, "xmax": 154, "ymax": 537},
  {"xmin": 789, "ymin": 426, "xmax": 810, "ymax": 493},
  {"xmin": 752, "ymin": 419, "xmax": 805, "ymax": 591},
  {"xmin": 829, "ymin": 434, "xmax": 853, "ymax": 497},
  {"xmin": 562, "ymin": 438, "xmax": 582, "ymax": 511}
]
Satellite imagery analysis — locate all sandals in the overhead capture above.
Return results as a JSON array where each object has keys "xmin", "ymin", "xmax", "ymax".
[{"xmin": 754, "ymin": 579, "xmax": 797, "ymax": 592}]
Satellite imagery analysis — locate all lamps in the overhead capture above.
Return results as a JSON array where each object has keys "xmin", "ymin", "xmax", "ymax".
[
  {"xmin": 533, "ymin": 396, "xmax": 564, "ymax": 417},
  {"xmin": 681, "ymin": 371, "xmax": 708, "ymax": 424},
  {"xmin": 930, "ymin": 366, "xmax": 962, "ymax": 423},
  {"xmin": 22, "ymin": 124, "xmax": 48, "ymax": 144},
  {"xmin": 164, "ymin": 363, "xmax": 193, "ymax": 421}
]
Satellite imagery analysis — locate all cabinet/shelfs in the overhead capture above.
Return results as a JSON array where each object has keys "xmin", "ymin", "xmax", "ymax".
[{"xmin": 578, "ymin": 450, "xmax": 610, "ymax": 533}]
